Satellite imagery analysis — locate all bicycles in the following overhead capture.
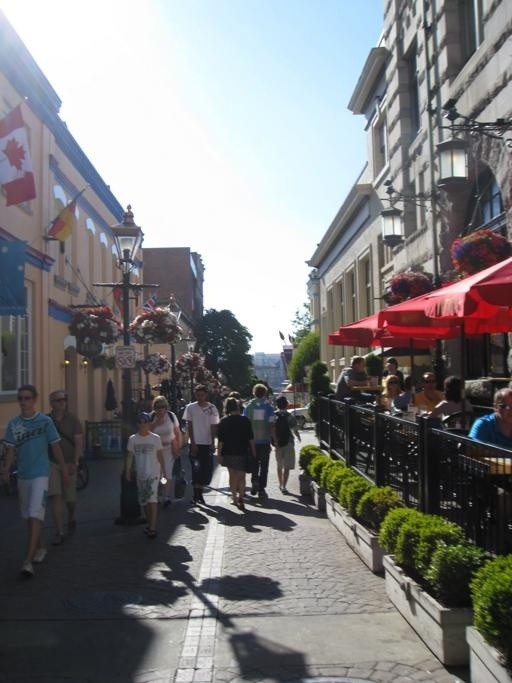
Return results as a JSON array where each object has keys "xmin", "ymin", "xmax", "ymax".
[{"xmin": 45, "ymin": 446, "xmax": 90, "ymax": 499}]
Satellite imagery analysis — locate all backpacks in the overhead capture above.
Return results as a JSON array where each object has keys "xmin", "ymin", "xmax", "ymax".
[{"xmin": 271, "ymin": 411, "xmax": 291, "ymax": 447}]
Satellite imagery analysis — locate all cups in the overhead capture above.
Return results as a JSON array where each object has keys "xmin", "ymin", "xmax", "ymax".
[
  {"xmin": 420, "ymin": 405, "xmax": 428, "ymax": 417},
  {"xmin": 360, "ymin": 382, "xmax": 364, "ymax": 388}
]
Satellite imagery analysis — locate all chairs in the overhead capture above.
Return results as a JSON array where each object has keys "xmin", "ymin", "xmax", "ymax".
[
  {"xmin": 348, "ymin": 417, "xmax": 373, "ymax": 473},
  {"xmin": 391, "ymin": 392, "xmax": 410, "ymax": 412},
  {"xmin": 442, "ymin": 411, "xmax": 480, "ymax": 437}
]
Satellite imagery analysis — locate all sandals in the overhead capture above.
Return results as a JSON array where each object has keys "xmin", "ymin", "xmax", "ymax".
[{"xmin": 147, "ymin": 529, "xmax": 157, "ymax": 537}]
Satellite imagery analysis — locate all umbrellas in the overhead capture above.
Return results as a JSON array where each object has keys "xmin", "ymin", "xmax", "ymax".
[
  {"xmin": 420, "ymin": 257, "xmax": 512, "ymax": 378},
  {"xmin": 378, "ymin": 288, "xmax": 510, "ymax": 374},
  {"xmin": 341, "ymin": 315, "xmax": 437, "ymax": 375},
  {"xmin": 328, "ymin": 331, "xmax": 436, "ymax": 385},
  {"xmin": 281, "ymin": 383, "xmax": 306, "ymax": 414}
]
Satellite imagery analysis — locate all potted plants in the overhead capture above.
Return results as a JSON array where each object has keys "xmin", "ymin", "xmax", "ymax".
[
  {"xmin": 309, "ymin": 457, "xmax": 343, "ymax": 510},
  {"xmin": 325, "ymin": 469, "xmax": 407, "ymax": 574},
  {"xmin": 299, "ymin": 445, "xmax": 323, "ymax": 495},
  {"xmin": 467, "ymin": 554, "xmax": 512, "ymax": 683},
  {"xmin": 383, "ymin": 505, "xmax": 485, "ymax": 666}
]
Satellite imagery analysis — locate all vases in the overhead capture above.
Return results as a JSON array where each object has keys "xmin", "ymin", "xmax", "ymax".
[
  {"xmin": 77, "ymin": 341, "xmax": 98, "ymax": 355},
  {"xmin": 400, "ymin": 297, "xmax": 411, "ymax": 302},
  {"xmin": 468, "ymin": 265, "xmax": 484, "ymax": 274}
]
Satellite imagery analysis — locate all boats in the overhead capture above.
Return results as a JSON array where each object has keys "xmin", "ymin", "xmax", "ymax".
[{"xmin": 161, "ymin": 295, "xmax": 181, "ymax": 415}]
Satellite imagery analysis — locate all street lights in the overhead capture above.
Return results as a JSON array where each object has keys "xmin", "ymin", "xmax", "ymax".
[{"xmin": 111, "ymin": 204, "xmax": 144, "ymax": 518}]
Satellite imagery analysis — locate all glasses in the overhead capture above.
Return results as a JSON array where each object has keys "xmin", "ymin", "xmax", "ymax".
[
  {"xmin": 389, "ymin": 382, "xmax": 398, "ymax": 384},
  {"xmin": 424, "ymin": 379, "xmax": 436, "ymax": 382},
  {"xmin": 494, "ymin": 403, "xmax": 512, "ymax": 411},
  {"xmin": 50, "ymin": 397, "xmax": 67, "ymax": 402},
  {"xmin": 18, "ymin": 395, "xmax": 33, "ymax": 401}
]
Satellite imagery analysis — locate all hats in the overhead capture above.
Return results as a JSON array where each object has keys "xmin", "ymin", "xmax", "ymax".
[{"xmin": 136, "ymin": 412, "xmax": 152, "ymax": 423}]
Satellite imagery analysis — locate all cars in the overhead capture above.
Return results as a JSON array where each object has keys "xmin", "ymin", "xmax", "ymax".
[{"xmin": 276, "ymin": 401, "xmax": 318, "ymax": 428}]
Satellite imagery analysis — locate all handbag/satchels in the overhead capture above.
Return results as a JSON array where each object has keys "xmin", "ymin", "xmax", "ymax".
[
  {"xmin": 246, "ymin": 419, "xmax": 257, "ymax": 473},
  {"xmin": 173, "ymin": 456, "xmax": 186, "ymax": 498},
  {"xmin": 49, "ymin": 414, "xmax": 84, "ymax": 469}
]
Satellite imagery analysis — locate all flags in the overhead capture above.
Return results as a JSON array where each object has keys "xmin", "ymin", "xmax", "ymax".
[
  {"xmin": 113, "ymin": 287, "xmax": 123, "ymax": 315},
  {"xmin": 143, "ymin": 294, "xmax": 155, "ymax": 310},
  {"xmin": 48, "ymin": 199, "xmax": 76, "ymax": 242},
  {"xmin": 0, "ymin": 96, "xmax": 36, "ymax": 207}
]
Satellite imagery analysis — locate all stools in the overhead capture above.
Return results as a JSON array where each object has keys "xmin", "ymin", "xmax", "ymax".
[{"xmin": 489, "ymin": 378, "xmax": 510, "ymax": 403}]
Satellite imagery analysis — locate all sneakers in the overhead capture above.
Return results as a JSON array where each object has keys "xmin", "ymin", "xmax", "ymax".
[
  {"xmin": 162, "ymin": 500, "xmax": 171, "ymax": 508},
  {"xmin": 236, "ymin": 502, "xmax": 244, "ymax": 509},
  {"xmin": 198, "ymin": 495, "xmax": 204, "ymax": 503},
  {"xmin": 51, "ymin": 533, "xmax": 65, "ymax": 546},
  {"xmin": 161, "ymin": 496, "xmax": 172, "ymax": 509},
  {"xmin": 20, "ymin": 561, "xmax": 35, "ymax": 575},
  {"xmin": 278, "ymin": 486, "xmax": 282, "ymax": 490},
  {"xmin": 251, "ymin": 488, "xmax": 258, "ymax": 495},
  {"xmin": 258, "ymin": 488, "xmax": 267, "ymax": 498},
  {"xmin": 32, "ymin": 549, "xmax": 47, "ymax": 562},
  {"xmin": 68, "ymin": 522, "xmax": 77, "ymax": 536},
  {"xmin": 191, "ymin": 496, "xmax": 196, "ymax": 507},
  {"xmin": 230, "ymin": 498, "xmax": 238, "ymax": 505},
  {"xmin": 281, "ymin": 488, "xmax": 288, "ymax": 494}
]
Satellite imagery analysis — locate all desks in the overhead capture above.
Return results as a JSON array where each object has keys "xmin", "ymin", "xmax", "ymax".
[{"xmin": 352, "ymin": 385, "xmax": 383, "ymax": 391}]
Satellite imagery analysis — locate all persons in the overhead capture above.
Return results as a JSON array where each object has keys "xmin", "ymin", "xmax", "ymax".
[
  {"xmin": 377, "ymin": 375, "xmax": 405, "ymax": 410},
  {"xmin": 0, "ymin": 385, "xmax": 77, "ymax": 576},
  {"xmin": 218, "ymin": 398, "xmax": 256, "ymax": 509},
  {"xmin": 413, "ymin": 372, "xmax": 445, "ymax": 411},
  {"xmin": 335, "ymin": 356, "xmax": 369, "ymax": 419},
  {"xmin": 428, "ymin": 375, "xmax": 473, "ymax": 429},
  {"xmin": 383, "ymin": 357, "xmax": 405, "ymax": 390},
  {"xmin": 271, "ymin": 397, "xmax": 302, "ymax": 495},
  {"xmin": 125, "ymin": 413, "xmax": 167, "ymax": 539},
  {"xmin": 242, "ymin": 384, "xmax": 275, "ymax": 500},
  {"xmin": 393, "ymin": 376, "xmax": 419, "ymax": 412},
  {"xmin": 148, "ymin": 396, "xmax": 182, "ymax": 509},
  {"xmin": 222, "ymin": 392, "xmax": 247, "ymax": 416},
  {"xmin": 467, "ymin": 388, "xmax": 512, "ymax": 449},
  {"xmin": 46, "ymin": 390, "xmax": 83, "ymax": 545},
  {"xmin": 181, "ymin": 385, "xmax": 220, "ymax": 507}
]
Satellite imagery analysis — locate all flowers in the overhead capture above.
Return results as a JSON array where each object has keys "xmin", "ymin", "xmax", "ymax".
[
  {"xmin": 175, "ymin": 351, "xmax": 204, "ymax": 375},
  {"xmin": 68, "ymin": 307, "xmax": 124, "ymax": 343},
  {"xmin": 195, "ymin": 366, "xmax": 214, "ymax": 384},
  {"xmin": 128, "ymin": 297, "xmax": 183, "ymax": 345},
  {"xmin": 390, "ymin": 272, "xmax": 433, "ymax": 300},
  {"xmin": 142, "ymin": 353, "xmax": 172, "ymax": 374},
  {"xmin": 450, "ymin": 229, "xmax": 512, "ymax": 271},
  {"xmin": 207, "ymin": 379, "xmax": 222, "ymax": 392}
]
